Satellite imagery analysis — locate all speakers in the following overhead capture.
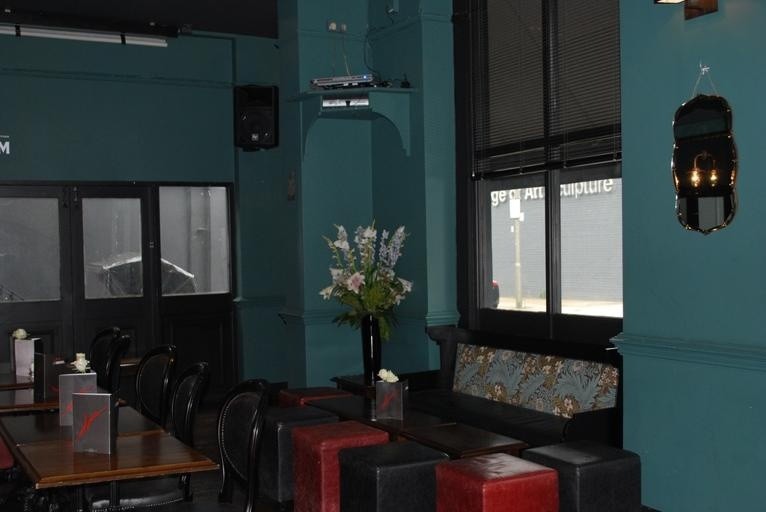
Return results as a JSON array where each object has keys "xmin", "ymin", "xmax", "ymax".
[{"xmin": 234, "ymin": 84, "xmax": 278, "ymax": 148}]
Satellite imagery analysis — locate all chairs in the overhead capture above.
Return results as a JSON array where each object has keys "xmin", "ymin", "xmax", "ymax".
[{"xmin": 79, "ymin": 326, "xmax": 278, "ymax": 511}]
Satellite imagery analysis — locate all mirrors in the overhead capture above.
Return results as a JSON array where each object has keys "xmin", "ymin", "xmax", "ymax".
[{"xmin": 672, "ymin": 93, "xmax": 738, "ymax": 235}]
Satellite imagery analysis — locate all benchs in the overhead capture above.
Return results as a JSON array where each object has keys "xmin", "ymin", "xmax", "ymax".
[{"xmin": 330, "ymin": 326, "xmax": 624, "ymax": 451}]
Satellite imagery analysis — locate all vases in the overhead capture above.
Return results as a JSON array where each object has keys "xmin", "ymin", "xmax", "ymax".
[{"xmin": 360, "ymin": 312, "xmax": 383, "ymax": 421}]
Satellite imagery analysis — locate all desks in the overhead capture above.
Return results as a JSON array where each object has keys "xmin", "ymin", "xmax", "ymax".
[{"xmin": 1, "ymin": 360, "xmax": 222, "ymax": 511}]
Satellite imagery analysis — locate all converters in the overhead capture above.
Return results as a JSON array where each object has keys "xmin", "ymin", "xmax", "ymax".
[{"xmin": 329, "ymin": 22, "xmax": 336, "ymax": 30}]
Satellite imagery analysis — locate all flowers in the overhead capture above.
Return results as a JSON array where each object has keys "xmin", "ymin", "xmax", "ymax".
[{"xmin": 317, "ymin": 215, "xmax": 415, "ymax": 344}]
